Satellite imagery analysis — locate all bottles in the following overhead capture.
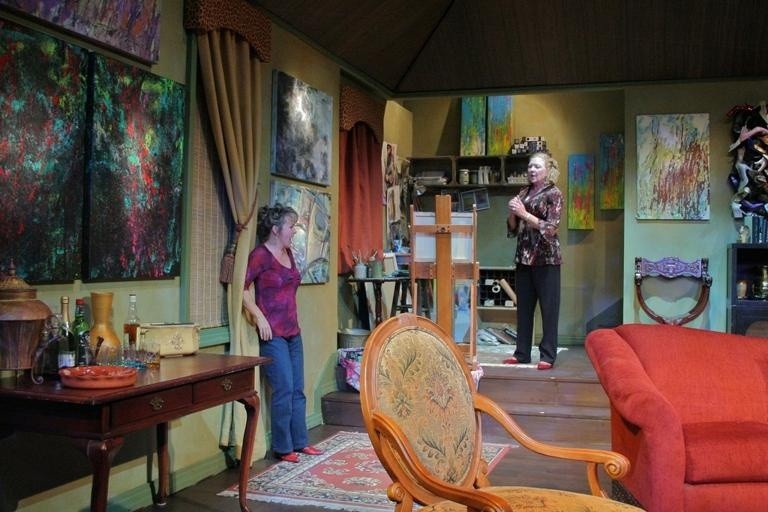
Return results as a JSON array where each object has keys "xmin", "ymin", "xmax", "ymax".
[
  {"xmin": 68, "ymin": 298, "xmax": 89, "ymax": 366},
  {"xmin": 123, "ymin": 294, "xmax": 142, "ymax": 347},
  {"xmin": 55, "ymin": 294, "xmax": 75, "ymax": 367}
]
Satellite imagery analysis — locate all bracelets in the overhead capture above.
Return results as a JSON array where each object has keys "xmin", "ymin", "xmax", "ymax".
[{"xmin": 524, "ymin": 212, "xmax": 532, "ymax": 221}]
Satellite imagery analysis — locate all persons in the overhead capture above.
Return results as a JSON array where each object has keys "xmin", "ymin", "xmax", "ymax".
[
  {"xmin": 241, "ymin": 202, "xmax": 327, "ymax": 463},
  {"xmin": 501, "ymin": 152, "xmax": 566, "ymax": 369}
]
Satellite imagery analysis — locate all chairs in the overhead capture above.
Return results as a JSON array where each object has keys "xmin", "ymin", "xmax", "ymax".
[{"xmin": 359, "ymin": 311, "xmax": 768, "ymax": 512}]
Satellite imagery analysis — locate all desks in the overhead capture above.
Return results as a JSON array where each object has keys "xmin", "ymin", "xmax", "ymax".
[
  {"xmin": 1, "ymin": 354, "xmax": 273, "ymax": 511},
  {"xmin": 349, "ymin": 276, "xmax": 410, "ymax": 333}
]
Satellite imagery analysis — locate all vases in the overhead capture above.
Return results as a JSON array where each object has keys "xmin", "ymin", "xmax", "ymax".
[{"xmin": 86, "ymin": 288, "xmax": 121, "ymax": 362}]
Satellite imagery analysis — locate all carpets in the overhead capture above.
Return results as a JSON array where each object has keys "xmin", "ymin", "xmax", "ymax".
[
  {"xmin": 457, "ymin": 340, "xmax": 569, "ymax": 369},
  {"xmin": 216, "ymin": 431, "xmax": 515, "ymax": 511}
]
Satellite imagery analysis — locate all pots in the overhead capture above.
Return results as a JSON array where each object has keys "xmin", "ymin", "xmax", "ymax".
[{"xmin": 141, "ymin": 321, "xmax": 201, "ymax": 358}]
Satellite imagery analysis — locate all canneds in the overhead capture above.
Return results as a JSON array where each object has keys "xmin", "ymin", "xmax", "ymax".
[{"xmin": 458, "ymin": 169, "xmax": 470, "ymax": 185}]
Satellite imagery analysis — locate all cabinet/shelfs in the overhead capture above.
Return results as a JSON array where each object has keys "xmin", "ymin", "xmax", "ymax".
[{"xmin": 406, "ymin": 153, "xmax": 538, "ymax": 187}]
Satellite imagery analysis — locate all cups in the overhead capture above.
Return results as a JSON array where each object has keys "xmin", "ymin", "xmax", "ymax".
[{"xmin": 91, "ymin": 344, "xmax": 160, "ymax": 370}]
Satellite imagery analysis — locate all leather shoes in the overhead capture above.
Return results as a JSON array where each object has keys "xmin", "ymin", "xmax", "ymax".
[
  {"xmin": 302, "ymin": 446, "xmax": 320, "ymax": 454},
  {"xmin": 280, "ymin": 453, "xmax": 299, "ymax": 462},
  {"xmin": 503, "ymin": 359, "xmax": 519, "ymax": 364},
  {"xmin": 538, "ymin": 362, "xmax": 553, "ymax": 370}
]
